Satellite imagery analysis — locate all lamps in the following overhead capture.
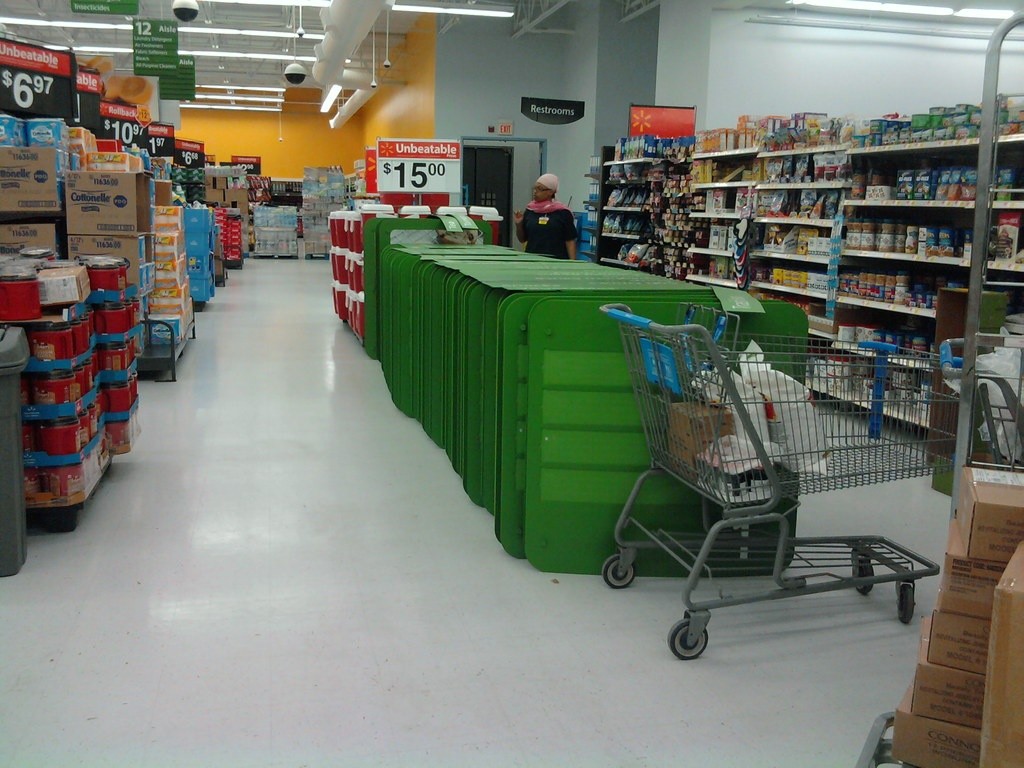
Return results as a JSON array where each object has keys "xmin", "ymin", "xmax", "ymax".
[
  {"xmin": 391, "ymin": 0, "xmax": 515, "ymax": 17},
  {"xmin": 0, "ymin": 0, "xmax": 352, "ymax": 63},
  {"xmin": 319, "ymin": 84, "xmax": 342, "ymax": 112},
  {"xmin": 180, "ymin": 84, "xmax": 286, "ymax": 112}
]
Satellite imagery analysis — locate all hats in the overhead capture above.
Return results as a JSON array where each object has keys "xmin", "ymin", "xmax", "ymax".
[{"xmin": 537, "ymin": 173, "xmax": 558, "ymax": 193}]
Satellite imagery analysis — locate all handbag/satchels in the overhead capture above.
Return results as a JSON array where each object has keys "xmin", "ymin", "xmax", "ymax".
[
  {"xmin": 737, "ymin": 338, "xmax": 831, "ymax": 477},
  {"xmin": 688, "ymin": 362, "xmax": 770, "ymax": 443},
  {"xmin": 696, "ymin": 434, "xmax": 785, "ymax": 474}
]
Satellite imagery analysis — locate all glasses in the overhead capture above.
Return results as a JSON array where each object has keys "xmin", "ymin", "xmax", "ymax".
[{"xmin": 533, "ymin": 185, "xmax": 552, "ymax": 192}]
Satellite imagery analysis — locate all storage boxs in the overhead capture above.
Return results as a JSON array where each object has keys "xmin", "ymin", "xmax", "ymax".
[
  {"xmin": 852, "ymin": 92, "xmax": 1024, "ymax": 201},
  {"xmin": 614, "ymin": 136, "xmax": 695, "ymax": 161},
  {"xmin": 0, "ymin": 145, "xmax": 347, "ymax": 497},
  {"xmin": 328, "ymin": 193, "xmax": 504, "ymax": 347},
  {"xmin": 666, "ymin": 398, "xmax": 732, "ymax": 482},
  {"xmin": 890, "ymin": 286, "xmax": 1024, "ymax": 768},
  {"xmin": 750, "ymin": 268, "xmax": 878, "ymax": 334},
  {"xmin": 692, "ymin": 112, "xmax": 827, "ymax": 280}
]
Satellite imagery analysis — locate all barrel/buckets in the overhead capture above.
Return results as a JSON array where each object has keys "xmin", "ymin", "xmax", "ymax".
[{"xmin": 327, "ymin": 204, "xmax": 503, "ymax": 337}]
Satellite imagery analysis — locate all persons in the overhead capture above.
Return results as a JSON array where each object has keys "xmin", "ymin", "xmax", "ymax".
[{"xmin": 514, "ymin": 174, "xmax": 578, "ymax": 260}]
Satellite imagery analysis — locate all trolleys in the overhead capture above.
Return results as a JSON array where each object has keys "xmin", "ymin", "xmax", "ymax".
[{"xmin": 597, "ymin": 303, "xmax": 1023, "ymax": 660}]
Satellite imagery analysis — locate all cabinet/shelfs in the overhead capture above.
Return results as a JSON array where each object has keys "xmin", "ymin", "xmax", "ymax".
[
  {"xmin": 578, "ymin": 132, "xmax": 1024, "ymax": 435},
  {"xmin": 0, "ymin": 284, "xmax": 143, "ymax": 532},
  {"xmin": 344, "ymin": 173, "xmax": 357, "ymax": 209},
  {"xmin": 270, "ymin": 190, "xmax": 303, "ymax": 238}
]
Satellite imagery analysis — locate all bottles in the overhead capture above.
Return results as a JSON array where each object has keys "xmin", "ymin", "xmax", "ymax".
[
  {"xmin": 846, "ymin": 218, "xmax": 907, "ymax": 252},
  {"xmin": 857, "ymin": 267, "xmax": 909, "ymax": 305}
]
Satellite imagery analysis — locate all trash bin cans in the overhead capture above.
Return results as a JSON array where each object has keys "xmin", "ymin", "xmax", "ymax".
[{"xmin": 0, "ymin": 325, "xmax": 29, "ymax": 578}]
